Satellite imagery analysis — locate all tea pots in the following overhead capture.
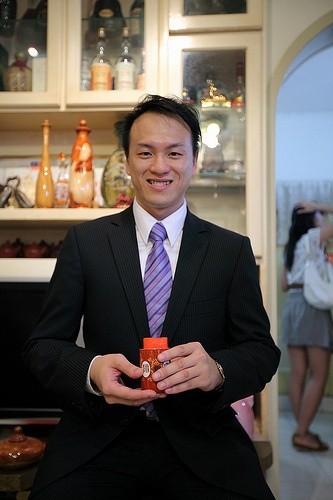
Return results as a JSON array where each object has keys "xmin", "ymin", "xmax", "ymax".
[
  {"xmin": 0, "ymin": 237, "xmax": 62, "ymax": 257},
  {"xmin": 0, "ymin": 426, "xmax": 44, "ymax": 468}
]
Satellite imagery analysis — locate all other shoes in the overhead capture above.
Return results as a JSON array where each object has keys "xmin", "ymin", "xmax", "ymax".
[{"xmin": 291, "ymin": 431, "xmax": 329, "ymax": 453}]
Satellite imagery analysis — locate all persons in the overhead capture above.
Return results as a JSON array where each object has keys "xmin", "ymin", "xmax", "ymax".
[
  {"xmin": 281, "ymin": 202, "xmax": 333, "ymax": 453},
  {"xmin": 22, "ymin": 93, "xmax": 280, "ymax": 500}
]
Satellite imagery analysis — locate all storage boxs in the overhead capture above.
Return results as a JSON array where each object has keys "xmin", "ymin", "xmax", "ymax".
[{"xmin": 139, "ymin": 348, "xmax": 170, "ymax": 394}]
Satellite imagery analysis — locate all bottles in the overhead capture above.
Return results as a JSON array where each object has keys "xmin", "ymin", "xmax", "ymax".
[
  {"xmin": 101, "ymin": 138, "xmax": 136, "ymax": 208},
  {"xmin": 72, "ymin": 121, "xmax": 95, "ymax": 208},
  {"xmin": 35, "ymin": 118, "xmax": 53, "ymax": 209},
  {"xmin": 230, "ymin": 62, "xmax": 245, "ymax": 108},
  {"xmin": 53, "ymin": 153, "xmax": 71, "ymax": 208},
  {"xmin": 0, "ymin": 0, "xmax": 145, "ymax": 92}
]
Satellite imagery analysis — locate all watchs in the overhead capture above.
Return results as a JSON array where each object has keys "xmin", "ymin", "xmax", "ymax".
[{"xmin": 213, "ymin": 360, "xmax": 225, "ymax": 390}]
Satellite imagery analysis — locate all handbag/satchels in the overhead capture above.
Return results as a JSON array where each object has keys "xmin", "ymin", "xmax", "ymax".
[{"xmin": 303, "ymin": 227, "xmax": 333, "ymax": 310}]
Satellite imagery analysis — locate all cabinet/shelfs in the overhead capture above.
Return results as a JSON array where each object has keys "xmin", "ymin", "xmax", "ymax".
[{"xmin": 0, "ymin": 0, "xmax": 273, "ymax": 282}]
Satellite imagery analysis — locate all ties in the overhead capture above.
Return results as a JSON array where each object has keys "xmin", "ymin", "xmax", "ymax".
[{"xmin": 144, "ymin": 221, "xmax": 173, "ymax": 413}]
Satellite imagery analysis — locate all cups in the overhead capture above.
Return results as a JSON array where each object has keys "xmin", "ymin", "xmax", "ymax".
[{"xmin": 140, "ymin": 349, "xmax": 171, "ymax": 394}]
